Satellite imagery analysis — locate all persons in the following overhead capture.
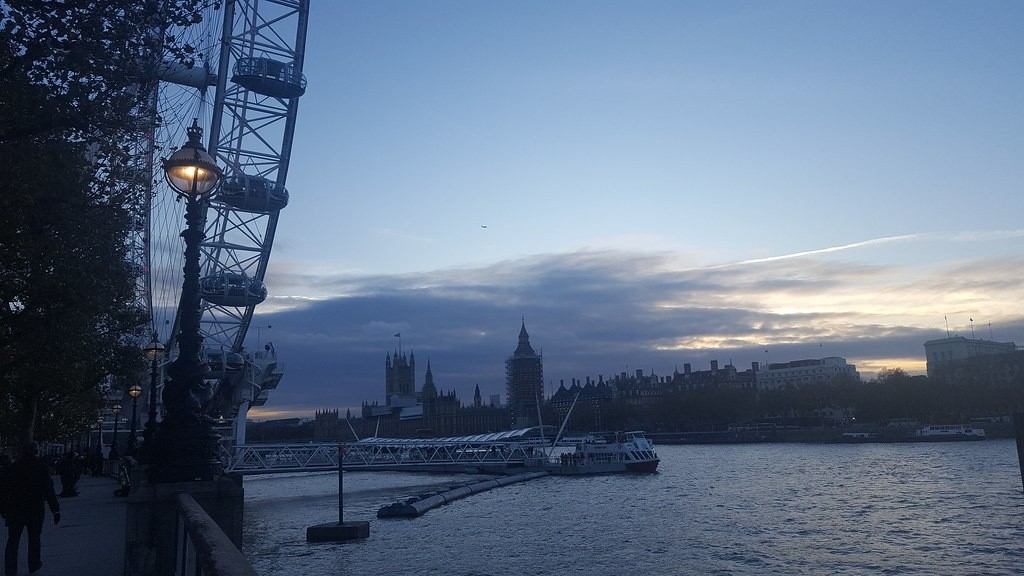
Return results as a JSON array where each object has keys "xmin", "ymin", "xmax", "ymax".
[{"xmin": 0, "ymin": 441, "xmax": 61, "ymax": 576}]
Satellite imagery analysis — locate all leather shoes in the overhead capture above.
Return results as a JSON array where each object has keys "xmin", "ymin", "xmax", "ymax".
[{"xmin": 29, "ymin": 560, "xmax": 43, "ymax": 574}]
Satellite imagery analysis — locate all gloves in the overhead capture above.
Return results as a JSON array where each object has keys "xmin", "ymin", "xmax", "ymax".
[{"xmin": 54, "ymin": 513, "xmax": 60, "ymax": 525}]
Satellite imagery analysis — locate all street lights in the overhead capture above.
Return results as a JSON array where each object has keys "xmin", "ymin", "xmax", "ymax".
[
  {"xmin": 158, "ymin": 115, "xmax": 224, "ymax": 482},
  {"xmin": 78, "ymin": 383, "xmax": 148, "ymax": 450},
  {"xmin": 144, "ymin": 329, "xmax": 168, "ymax": 455}
]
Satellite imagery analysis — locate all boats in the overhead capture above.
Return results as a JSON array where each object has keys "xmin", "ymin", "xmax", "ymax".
[{"xmin": 576, "ymin": 428, "xmax": 661, "ymax": 476}]
